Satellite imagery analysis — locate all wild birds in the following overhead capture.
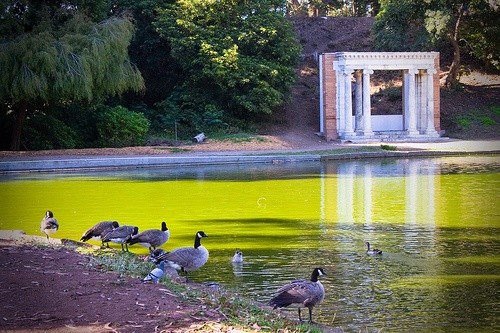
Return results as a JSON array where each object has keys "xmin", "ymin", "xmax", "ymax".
[
  {"xmin": 39, "ymin": 210, "xmax": 59, "ymax": 242},
  {"xmin": 232, "ymin": 249, "xmax": 244, "ymax": 264},
  {"xmin": 267, "ymin": 267, "xmax": 328, "ymax": 324},
  {"xmin": 101, "ymin": 225, "xmax": 140, "ymax": 258},
  {"xmin": 125, "ymin": 220, "xmax": 170, "ymax": 259},
  {"xmin": 363, "ymin": 241, "xmax": 383, "ymax": 257},
  {"xmin": 79, "ymin": 219, "xmax": 120, "ymax": 249},
  {"xmin": 159, "ymin": 231, "xmax": 210, "ymax": 284}
]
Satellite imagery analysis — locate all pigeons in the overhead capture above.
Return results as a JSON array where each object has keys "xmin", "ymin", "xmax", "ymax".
[{"xmin": 142, "ymin": 260, "xmax": 167, "ymax": 285}]
